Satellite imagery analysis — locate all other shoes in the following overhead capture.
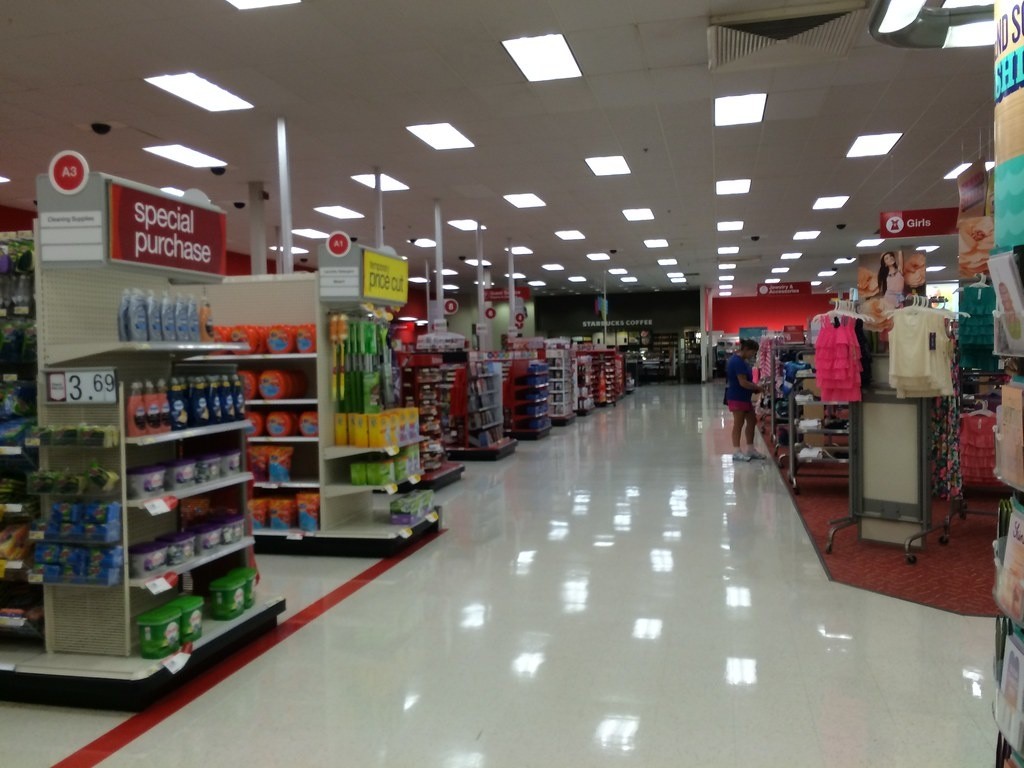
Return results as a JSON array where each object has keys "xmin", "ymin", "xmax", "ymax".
[
  {"xmin": 733, "ymin": 453, "xmax": 751, "ymax": 461},
  {"xmin": 746, "ymin": 450, "xmax": 767, "ymax": 459}
]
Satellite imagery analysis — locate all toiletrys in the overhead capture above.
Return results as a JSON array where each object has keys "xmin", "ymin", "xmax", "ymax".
[
  {"xmin": 127, "ymin": 374, "xmax": 246, "ymax": 439},
  {"xmin": 117, "ymin": 287, "xmax": 215, "ymax": 344}
]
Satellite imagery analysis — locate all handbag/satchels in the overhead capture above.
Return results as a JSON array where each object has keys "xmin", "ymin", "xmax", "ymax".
[{"xmin": 723, "ymin": 387, "xmax": 729, "ymax": 405}]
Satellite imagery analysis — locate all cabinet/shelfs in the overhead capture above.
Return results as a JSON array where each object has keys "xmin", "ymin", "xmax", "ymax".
[
  {"xmin": 401, "ymin": 341, "xmax": 700, "ymax": 494},
  {"xmin": 195, "ymin": 270, "xmax": 442, "ymax": 563},
  {"xmin": 333, "ymin": 407, "xmax": 434, "ymax": 525},
  {"xmin": 0, "ymin": 230, "xmax": 287, "ymax": 712},
  {"xmin": 765, "ymin": 334, "xmax": 849, "ymax": 498}
]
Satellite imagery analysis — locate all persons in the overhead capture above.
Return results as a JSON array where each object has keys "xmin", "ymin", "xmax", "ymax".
[
  {"xmin": 726, "ymin": 339, "xmax": 766, "ymax": 461},
  {"xmin": 877, "ymin": 250, "xmax": 904, "ymax": 310}
]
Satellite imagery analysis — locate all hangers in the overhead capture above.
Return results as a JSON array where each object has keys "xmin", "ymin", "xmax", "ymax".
[
  {"xmin": 761, "ymin": 330, "xmax": 783, "ymax": 338},
  {"xmin": 960, "ymin": 400, "xmax": 995, "ymax": 418},
  {"xmin": 812, "ymin": 300, "xmax": 875, "ymax": 323},
  {"xmin": 881, "ymin": 294, "xmax": 971, "ymax": 320}
]
